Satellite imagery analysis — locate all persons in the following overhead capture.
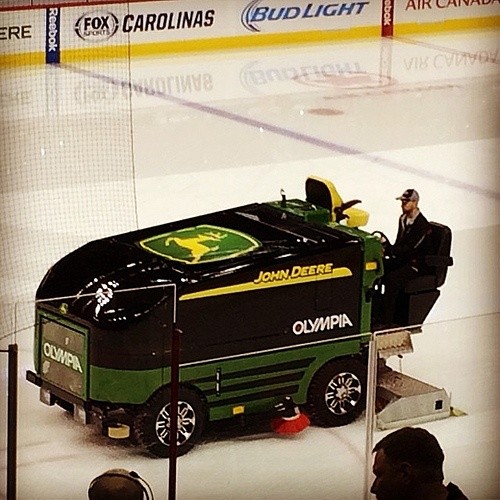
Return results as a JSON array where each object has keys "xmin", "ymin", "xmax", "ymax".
[
  {"xmin": 373, "ymin": 189, "xmax": 435, "ymax": 330},
  {"xmin": 369, "ymin": 427, "xmax": 469, "ymax": 500},
  {"xmin": 88, "ymin": 469, "xmax": 143, "ymax": 500}
]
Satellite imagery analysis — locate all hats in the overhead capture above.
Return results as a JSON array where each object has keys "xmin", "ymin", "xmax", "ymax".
[{"xmin": 396, "ymin": 189, "xmax": 419, "ymax": 201}]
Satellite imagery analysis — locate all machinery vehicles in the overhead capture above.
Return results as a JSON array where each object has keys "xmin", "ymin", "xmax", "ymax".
[{"xmin": 24, "ymin": 175, "xmax": 453, "ymax": 458}]
